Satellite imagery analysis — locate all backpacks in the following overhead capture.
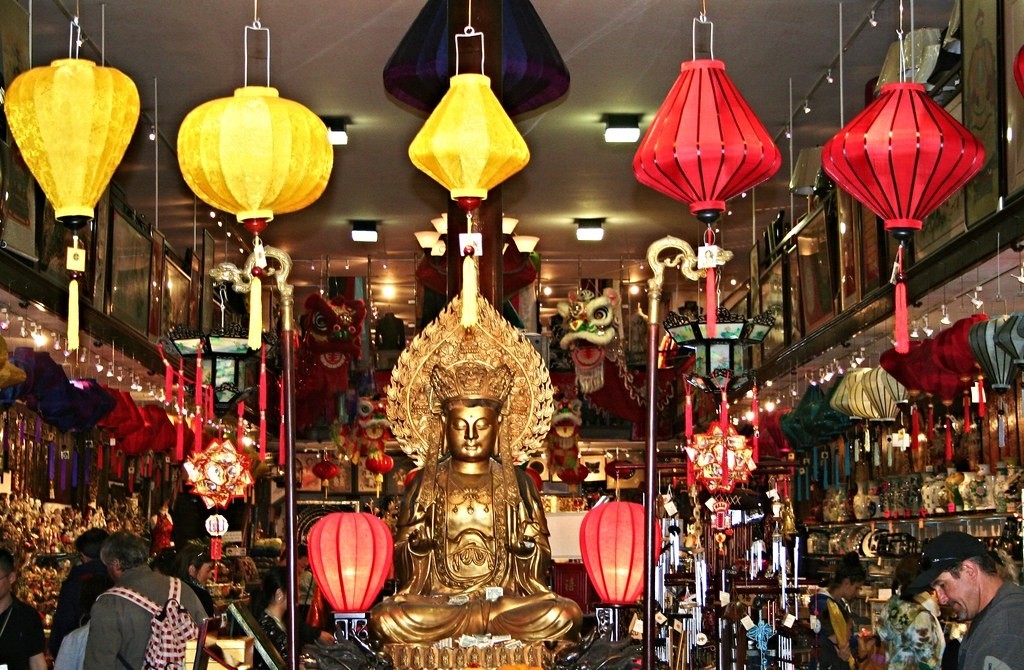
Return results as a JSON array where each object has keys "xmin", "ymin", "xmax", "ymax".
[{"xmin": 96, "ymin": 576, "xmax": 200, "ymax": 670}]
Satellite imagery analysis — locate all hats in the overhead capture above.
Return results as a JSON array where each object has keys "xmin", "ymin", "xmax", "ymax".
[
  {"xmin": 896, "ymin": 553, "xmax": 930, "ymax": 598},
  {"xmin": 919, "ymin": 530, "xmax": 989, "ymax": 584}
]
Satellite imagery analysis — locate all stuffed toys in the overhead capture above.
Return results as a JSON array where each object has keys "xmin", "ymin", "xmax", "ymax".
[
  {"xmin": 556, "ymin": 288, "xmax": 672, "ymax": 440},
  {"xmin": 280, "ymin": 292, "xmax": 367, "ymax": 430}
]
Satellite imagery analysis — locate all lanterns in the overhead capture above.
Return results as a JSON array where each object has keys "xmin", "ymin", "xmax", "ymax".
[
  {"xmin": 578, "ymin": 501, "xmax": 662, "ymax": 639},
  {"xmin": 183, "ymin": 436, "xmax": 255, "ymax": 581},
  {"xmin": 631, "ymin": 59, "xmax": 782, "ymax": 337},
  {"xmin": 177, "ymin": 86, "xmax": 334, "ymax": 351},
  {"xmin": 754, "ymin": 312, "xmax": 1024, "ymax": 503},
  {"xmin": 663, "ymin": 306, "xmax": 779, "ymax": 434},
  {"xmin": 311, "ymin": 453, "xmax": 426, "ymax": 495},
  {"xmin": 525, "ymin": 460, "xmax": 636, "ymax": 492},
  {"xmin": 683, "ymin": 420, "xmax": 757, "ymax": 555},
  {"xmin": 0, "ymin": 336, "xmax": 272, "ymax": 507},
  {"xmin": 4, "ymin": 59, "xmax": 141, "ymax": 349},
  {"xmin": 821, "ymin": 81, "xmax": 985, "ymax": 354},
  {"xmin": 307, "ymin": 510, "xmax": 395, "ymax": 642},
  {"xmin": 407, "ymin": 73, "xmax": 531, "ymax": 327},
  {"xmin": 1013, "ymin": 44, "xmax": 1024, "ymax": 98}
]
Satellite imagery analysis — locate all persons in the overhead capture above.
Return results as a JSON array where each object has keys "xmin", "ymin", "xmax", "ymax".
[
  {"xmin": 151, "ymin": 507, "xmax": 174, "ymax": 557},
  {"xmin": 368, "ymin": 291, "xmax": 582, "ymax": 645},
  {"xmin": 0, "ymin": 528, "xmax": 336, "ymax": 670},
  {"xmin": 808, "ymin": 532, "xmax": 1024, "ymax": 670}
]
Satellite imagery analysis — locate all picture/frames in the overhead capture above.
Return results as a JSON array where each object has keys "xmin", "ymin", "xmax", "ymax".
[
  {"xmin": 727, "ymin": 0, "xmax": 1024, "ymax": 388},
  {"xmin": 0, "ymin": 117, "xmax": 192, "ymax": 364}
]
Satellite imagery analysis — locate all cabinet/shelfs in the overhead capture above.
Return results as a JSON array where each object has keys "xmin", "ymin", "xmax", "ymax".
[{"xmin": 803, "ymin": 508, "xmax": 1022, "ymax": 560}]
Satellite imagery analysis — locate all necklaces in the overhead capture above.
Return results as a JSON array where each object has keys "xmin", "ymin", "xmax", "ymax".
[
  {"xmin": 0, "ymin": 605, "xmax": 13, "ymax": 637},
  {"xmin": 272, "ymin": 616, "xmax": 281, "ymax": 623}
]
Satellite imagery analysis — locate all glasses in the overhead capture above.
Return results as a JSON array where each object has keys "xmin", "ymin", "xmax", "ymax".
[
  {"xmin": 198, "ymin": 545, "xmax": 208, "ymax": 557},
  {"xmin": 919, "ymin": 552, "xmax": 958, "ymax": 570}
]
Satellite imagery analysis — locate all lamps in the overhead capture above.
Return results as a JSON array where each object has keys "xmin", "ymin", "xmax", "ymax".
[
  {"xmin": 351, "ymin": 222, "xmax": 377, "ymax": 242},
  {"xmin": 413, "ymin": 210, "xmax": 540, "ymax": 277},
  {"xmin": 604, "ymin": 117, "xmax": 640, "ymax": 142},
  {"xmin": 574, "ymin": 218, "xmax": 606, "ymax": 241},
  {"xmin": 322, "ymin": 119, "xmax": 349, "ymax": 146}
]
